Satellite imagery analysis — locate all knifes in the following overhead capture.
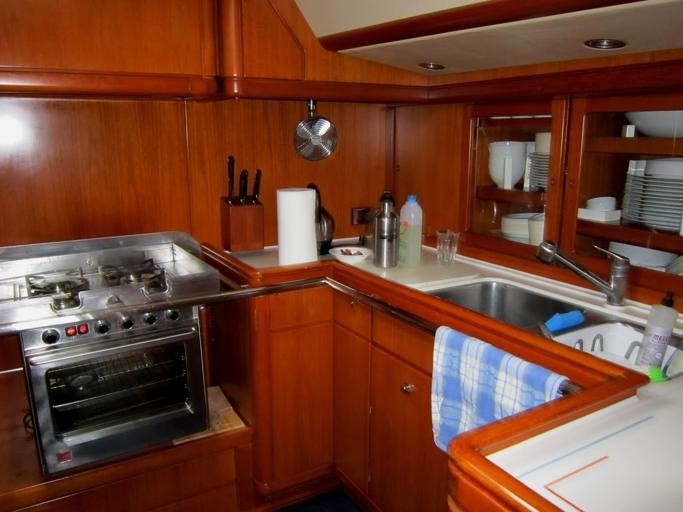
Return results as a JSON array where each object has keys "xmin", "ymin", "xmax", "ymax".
[{"xmin": 224, "ymin": 154, "xmax": 262, "ymax": 206}]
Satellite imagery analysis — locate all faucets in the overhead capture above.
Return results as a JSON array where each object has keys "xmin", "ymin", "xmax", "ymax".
[{"xmin": 534, "ymin": 239, "xmax": 630, "ymax": 304}]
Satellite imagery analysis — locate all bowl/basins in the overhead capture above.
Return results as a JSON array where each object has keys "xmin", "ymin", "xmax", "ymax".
[
  {"xmin": 328, "ymin": 245, "xmax": 372, "ymax": 264},
  {"xmin": 585, "ymin": 195, "xmax": 618, "ymax": 214},
  {"xmin": 625, "ymin": 109, "xmax": 683, "ymax": 139},
  {"xmin": 607, "ymin": 241, "xmax": 679, "ymax": 268}
]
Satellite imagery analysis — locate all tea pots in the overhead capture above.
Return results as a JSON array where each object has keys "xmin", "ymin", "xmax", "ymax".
[{"xmin": 306, "ymin": 182, "xmax": 335, "ymax": 255}]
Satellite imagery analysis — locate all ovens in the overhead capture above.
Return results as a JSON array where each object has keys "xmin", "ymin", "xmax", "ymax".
[{"xmin": 18, "ymin": 299, "xmax": 212, "ymax": 484}]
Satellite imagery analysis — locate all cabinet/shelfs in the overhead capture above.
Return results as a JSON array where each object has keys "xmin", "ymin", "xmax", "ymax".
[
  {"xmin": 369, "ymin": 310, "xmax": 459, "ymax": 512},
  {"xmin": 0, "ymin": 94, "xmax": 392, "ymax": 261},
  {"xmin": 390, "ymin": 104, "xmax": 473, "ymax": 239},
  {"xmin": 464, "ymin": 101, "xmax": 564, "ymax": 260},
  {"xmin": 332, "ymin": 294, "xmax": 371, "ymax": 500},
  {"xmin": 563, "ymin": 94, "xmax": 682, "ymax": 288},
  {"xmin": 256, "ymin": 291, "xmax": 334, "ymax": 485}
]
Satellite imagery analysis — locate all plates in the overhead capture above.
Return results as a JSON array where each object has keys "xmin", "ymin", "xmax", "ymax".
[
  {"xmin": 527, "ymin": 151, "xmax": 551, "ymax": 190},
  {"xmin": 500, "ymin": 212, "xmax": 544, "ymax": 247},
  {"xmin": 621, "ymin": 155, "xmax": 683, "ymax": 233}
]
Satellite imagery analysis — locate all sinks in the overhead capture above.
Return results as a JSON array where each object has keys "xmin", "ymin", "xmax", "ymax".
[
  {"xmin": 408, "ymin": 271, "xmax": 587, "ymax": 329},
  {"xmin": 532, "ymin": 317, "xmax": 683, "ymax": 378}
]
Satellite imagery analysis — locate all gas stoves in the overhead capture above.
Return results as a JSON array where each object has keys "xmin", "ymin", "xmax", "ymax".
[{"xmin": 0, "ymin": 238, "xmax": 221, "ymax": 325}]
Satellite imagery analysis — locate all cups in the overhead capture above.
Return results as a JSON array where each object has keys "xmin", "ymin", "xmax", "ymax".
[
  {"xmin": 486, "ymin": 140, "xmax": 528, "ymax": 189},
  {"xmin": 436, "ymin": 228, "xmax": 461, "ymax": 266}
]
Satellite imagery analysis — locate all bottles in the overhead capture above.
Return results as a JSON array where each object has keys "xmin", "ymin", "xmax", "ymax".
[
  {"xmin": 399, "ymin": 194, "xmax": 424, "ymax": 269},
  {"xmin": 372, "ymin": 192, "xmax": 398, "ymax": 268},
  {"xmin": 634, "ymin": 290, "xmax": 678, "ymax": 371}
]
